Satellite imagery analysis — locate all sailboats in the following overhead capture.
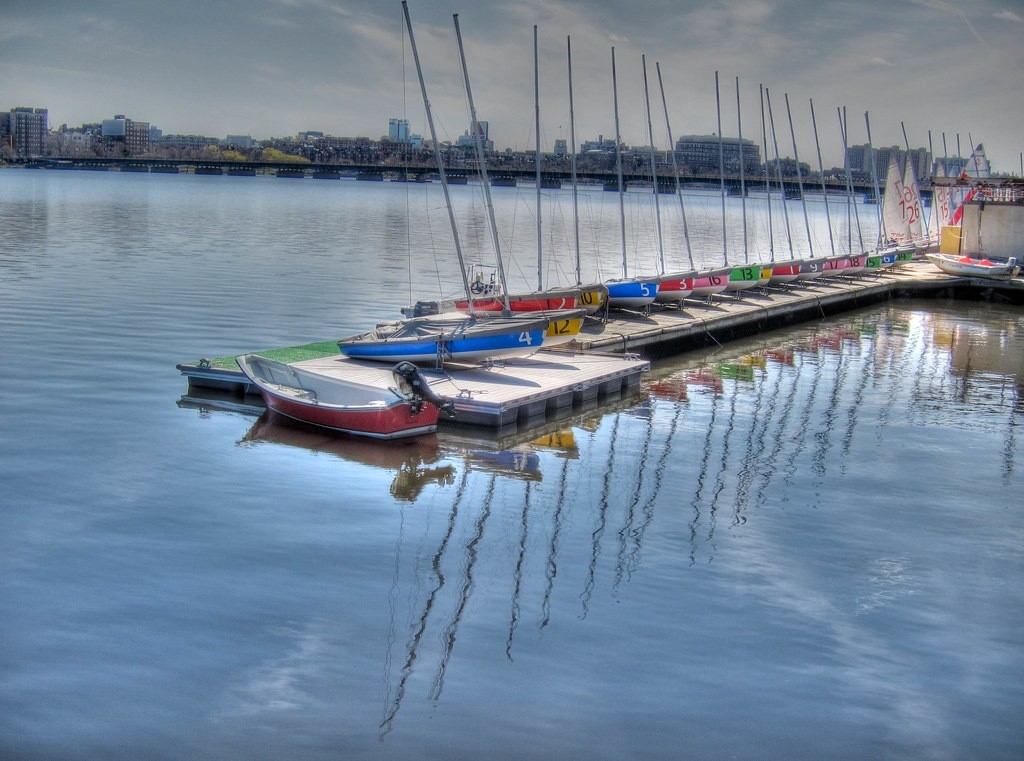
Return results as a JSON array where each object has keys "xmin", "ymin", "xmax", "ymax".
[
  {"xmin": 380, "ymin": 303, "xmax": 914, "ymax": 747},
  {"xmin": 334, "ymin": 0, "xmax": 938, "ymax": 366}
]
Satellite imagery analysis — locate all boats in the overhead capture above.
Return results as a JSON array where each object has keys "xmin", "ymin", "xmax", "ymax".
[
  {"xmin": 922, "ymin": 249, "xmax": 1016, "ymax": 279},
  {"xmin": 235, "ymin": 350, "xmax": 441, "ymax": 444}
]
